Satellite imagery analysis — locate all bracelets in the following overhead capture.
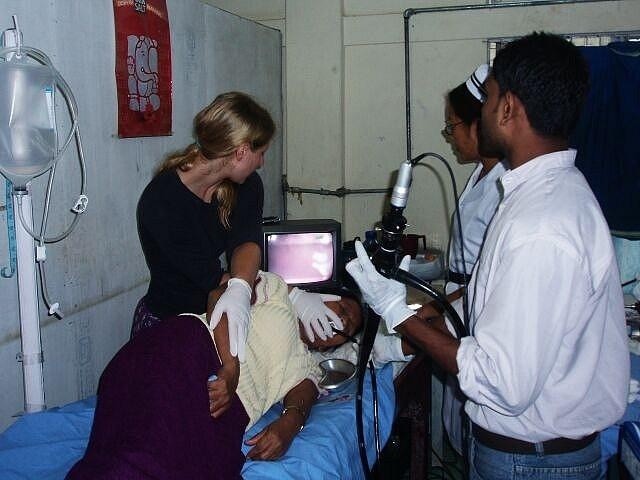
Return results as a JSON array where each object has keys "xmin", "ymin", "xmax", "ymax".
[
  {"xmin": 418, "ymin": 305, "xmax": 424, "ymax": 317},
  {"xmin": 280, "ymin": 405, "xmax": 310, "ymax": 425}
]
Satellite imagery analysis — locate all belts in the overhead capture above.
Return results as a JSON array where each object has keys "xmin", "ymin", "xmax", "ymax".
[{"xmin": 463, "ymin": 412, "xmax": 605, "ymax": 459}]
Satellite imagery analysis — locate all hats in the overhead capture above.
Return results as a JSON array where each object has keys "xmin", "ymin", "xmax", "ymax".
[{"xmin": 466, "ymin": 62, "xmax": 496, "ymax": 106}]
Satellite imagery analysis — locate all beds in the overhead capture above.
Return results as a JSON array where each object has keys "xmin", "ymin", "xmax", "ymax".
[{"xmin": 0, "ymin": 325, "xmax": 418, "ymax": 480}]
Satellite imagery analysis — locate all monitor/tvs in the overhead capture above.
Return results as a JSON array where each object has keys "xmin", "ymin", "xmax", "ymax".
[{"xmin": 259, "ymin": 218, "xmax": 342, "ymax": 292}]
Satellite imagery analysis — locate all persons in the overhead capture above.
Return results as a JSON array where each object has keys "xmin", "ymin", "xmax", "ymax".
[
  {"xmin": 346, "ymin": 30, "xmax": 629, "ymax": 479},
  {"xmin": 129, "ymin": 91, "xmax": 277, "ymax": 363},
  {"xmin": 63, "ymin": 271, "xmax": 366, "ymax": 480},
  {"xmin": 370, "ymin": 63, "xmax": 511, "ymax": 478}
]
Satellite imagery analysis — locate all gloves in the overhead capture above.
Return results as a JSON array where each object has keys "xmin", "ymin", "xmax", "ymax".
[
  {"xmin": 343, "ymin": 239, "xmax": 421, "ymax": 336},
  {"xmin": 207, "ymin": 275, "xmax": 254, "ymax": 366},
  {"xmin": 286, "ymin": 285, "xmax": 347, "ymax": 344}
]
